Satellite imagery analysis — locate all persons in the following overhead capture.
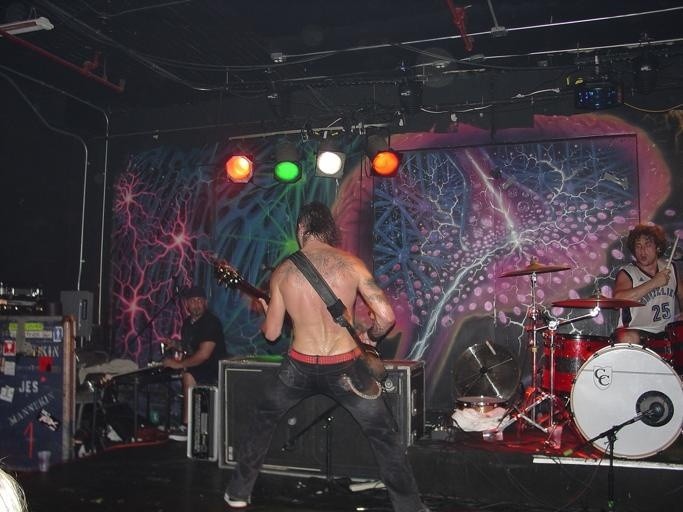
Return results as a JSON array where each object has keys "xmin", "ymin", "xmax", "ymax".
[
  {"xmin": 157, "ymin": 286, "xmax": 226, "ymax": 444},
  {"xmin": 608, "ymin": 222, "xmax": 682, "ymax": 347},
  {"xmin": 220, "ymin": 198, "xmax": 430, "ymax": 512}
]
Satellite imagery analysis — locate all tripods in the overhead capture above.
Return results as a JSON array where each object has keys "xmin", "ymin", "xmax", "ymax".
[
  {"xmin": 279, "ymin": 399, "xmax": 368, "ymax": 500},
  {"xmin": 501, "ymin": 274, "xmax": 564, "ymax": 435},
  {"xmin": 498, "ymin": 326, "xmax": 573, "ymax": 436}
]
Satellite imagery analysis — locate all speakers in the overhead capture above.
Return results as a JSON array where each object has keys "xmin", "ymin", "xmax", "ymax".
[{"xmin": 187, "ymin": 384, "xmax": 219, "ymax": 463}]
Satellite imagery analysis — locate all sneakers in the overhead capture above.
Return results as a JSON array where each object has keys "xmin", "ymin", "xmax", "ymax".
[
  {"xmin": 158, "ymin": 423, "xmax": 187, "ymax": 441},
  {"xmin": 224, "ymin": 493, "xmax": 247, "ymax": 508}
]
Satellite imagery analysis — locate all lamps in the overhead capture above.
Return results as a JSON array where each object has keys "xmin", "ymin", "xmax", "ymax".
[{"xmin": 223, "ymin": 122, "xmax": 402, "ymax": 185}]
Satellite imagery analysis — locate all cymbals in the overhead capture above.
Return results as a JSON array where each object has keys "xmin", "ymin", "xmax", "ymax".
[
  {"xmin": 551, "ymin": 295, "xmax": 646, "ymax": 308},
  {"xmin": 498, "ymin": 264, "xmax": 572, "ymax": 278},
  {"xmin": 450, "ymin": 341, "xmax": 522, "ymax": 402}
]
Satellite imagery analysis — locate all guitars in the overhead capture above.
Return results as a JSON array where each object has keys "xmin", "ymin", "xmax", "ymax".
[{"xmin": 212, "ymin": 257, "xmax": 386, "ymax": 385}]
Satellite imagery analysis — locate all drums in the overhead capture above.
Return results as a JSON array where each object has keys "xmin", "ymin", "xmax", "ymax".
[
  {"xmin": 456, "ymin": 397, "xmax": 508, "ymax": 414},
  {"xmin": 645, "ymin": 332, "xmax": 671, "ymax": 361},
  {"xmin": 566, "ymin": 341, "xmax": 682, "ymax": 459},
  {"xmin": 541, "ymin": 332, "xmax": 611, "ymax": 392},
  {"xmin": 668, "ymin": 321, "xmax": 682, "ymax": 371}
]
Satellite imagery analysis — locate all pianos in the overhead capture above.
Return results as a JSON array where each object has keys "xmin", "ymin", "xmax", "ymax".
[{"xmin": 111, "ymin": 366, "xmax": 183, "ymax": 385}]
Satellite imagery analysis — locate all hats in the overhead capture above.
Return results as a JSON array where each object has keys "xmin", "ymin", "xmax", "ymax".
[{"xmin": 187, "ymin": 286, "xmax": 205, "ymax": 299}]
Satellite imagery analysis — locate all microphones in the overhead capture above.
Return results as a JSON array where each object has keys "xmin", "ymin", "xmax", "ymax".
[
  {"xmin": 174, "ymin": 271, "xmax": 187, "ymax": 298},
  {"xmin": 639, "ymin": 403, "xmax": 667, "ymax": 424}
]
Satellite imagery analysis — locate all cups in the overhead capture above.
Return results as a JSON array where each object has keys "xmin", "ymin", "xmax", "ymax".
[
  {"xmin": 547, "ymin": 426, "xmax": 562, "ymax": 450},
  {"xmin": 38, "ymin": 450, "xmax": 51, "ymax": 472},
  {"xmin": 482, "ymin": 429, "xmax": 504, "ymax": 441}
]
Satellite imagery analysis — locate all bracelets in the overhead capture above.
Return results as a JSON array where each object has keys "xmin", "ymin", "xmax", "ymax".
[{"xmin": 366, "ymin": 329, "xmax": 376, "ymax": 342}]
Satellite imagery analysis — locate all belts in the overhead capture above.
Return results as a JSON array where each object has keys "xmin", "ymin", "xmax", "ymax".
[{"xmin": 290, "ymin": 347, "xmax": 362, "ymax": 365}]
